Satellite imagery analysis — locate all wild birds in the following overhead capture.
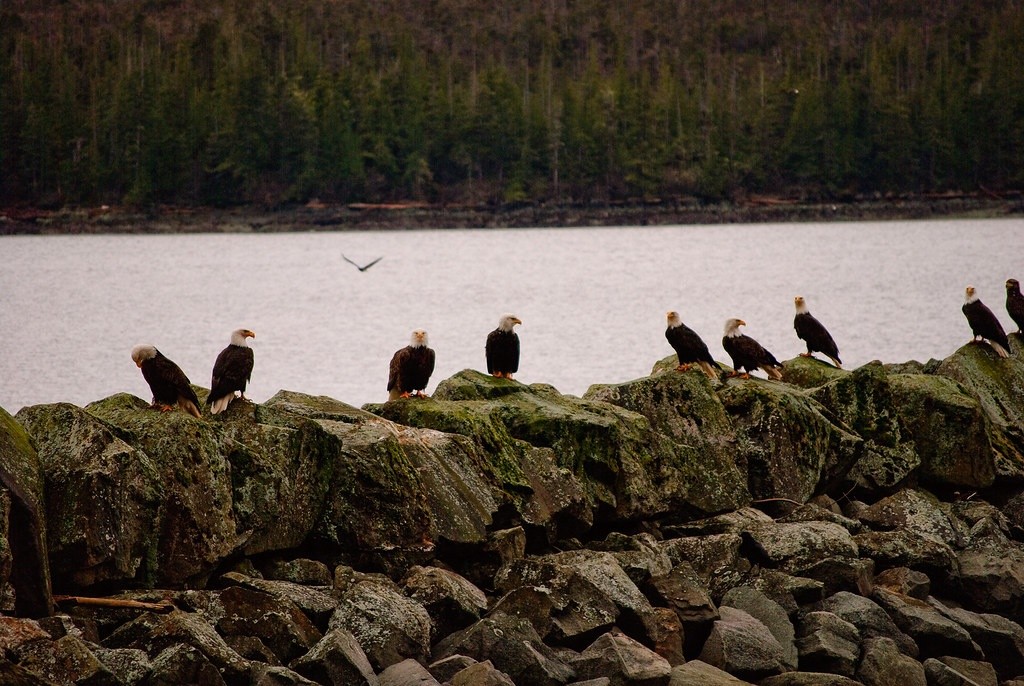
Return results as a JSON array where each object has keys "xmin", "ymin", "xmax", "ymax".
[{"xmin": 341, "ymin": 252, "xmax": 383, "ymax": 273}]
